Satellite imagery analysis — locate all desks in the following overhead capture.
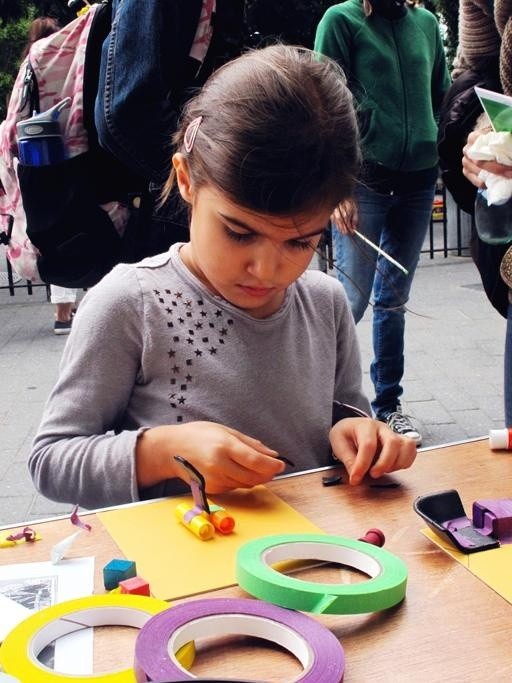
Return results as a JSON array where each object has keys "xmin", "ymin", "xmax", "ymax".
[{"xmin": 0, "ymin": 429, "xmax": 512, "ymax": 682}]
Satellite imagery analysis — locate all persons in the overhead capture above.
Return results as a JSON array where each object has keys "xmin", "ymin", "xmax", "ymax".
[
  {"xmin": 14, "ymin": 0, "xmax": 512, "ymax": 449},
  {"xmin": 29, "ymin": 44, "xmax": 418, "ymax": 509}
]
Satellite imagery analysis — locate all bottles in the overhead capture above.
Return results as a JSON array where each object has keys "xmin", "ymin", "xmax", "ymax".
[{"xmin": 15, "ymin": 95, "xmax": 76, "ymax": 283}]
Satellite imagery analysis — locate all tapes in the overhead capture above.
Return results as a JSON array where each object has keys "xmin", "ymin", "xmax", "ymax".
[
  {"xmin": 136, "ymin": 597, "xmax": 344, "ymax": 683},
  {"xmin": 237, "ymin": 533, "xmax": 410, "ymax": 615},
  {"xmin": 1, "ymin": 592, "xmax": 196, "ymax": 683}
]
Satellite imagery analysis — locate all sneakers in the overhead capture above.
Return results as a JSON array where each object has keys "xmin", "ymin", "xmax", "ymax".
[
  {"xmin": 379, "ymin": 406, "xmax": 422, "ymax": 447},
  {"xmin": 55, "ymin": 318, "xmax": 72, "ymax": 334}
]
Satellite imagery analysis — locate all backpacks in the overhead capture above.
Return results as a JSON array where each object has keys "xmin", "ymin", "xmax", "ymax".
[{"xmin": 0, "ymin": 1, "xmax": 134, "ymax": 290}]
